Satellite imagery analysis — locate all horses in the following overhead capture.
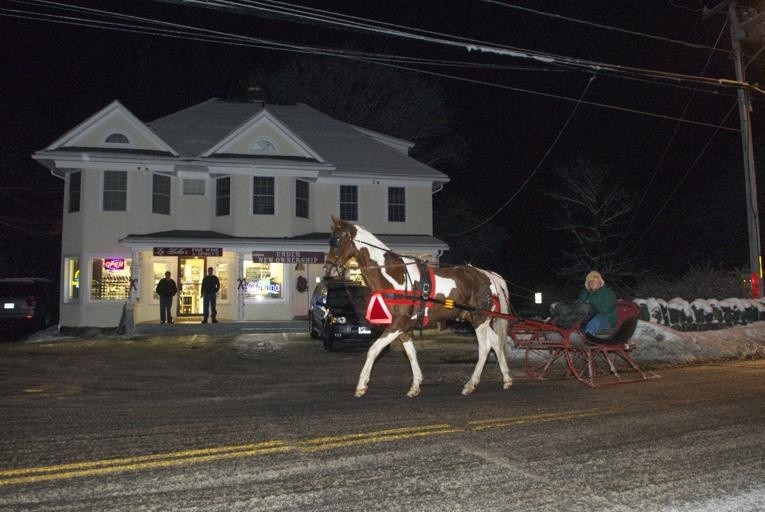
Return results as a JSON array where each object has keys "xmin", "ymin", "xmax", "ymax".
[{"xmin": 322, "ymin": 213, "xmax": 514, "ymax": 398}]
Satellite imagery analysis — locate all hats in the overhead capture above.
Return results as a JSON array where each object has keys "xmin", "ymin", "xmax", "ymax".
[{"xmin": 585, "ymin": 270, "xmax": 605, "ymax": 290}]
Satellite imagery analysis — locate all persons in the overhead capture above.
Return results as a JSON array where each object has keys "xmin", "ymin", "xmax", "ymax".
[
  {"xmin": 155, "ymin": 270, "xmax": 177, "ymax": 324},
  {"xmin": 200, "ymin": 266, "xmax": 220, "ymax": 324},
  {"xmin": 574, "ymin": 270, "xmax": 618, "ymax": 335}
]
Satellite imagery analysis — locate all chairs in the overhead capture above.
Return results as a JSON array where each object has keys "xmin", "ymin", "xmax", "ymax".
[{"xmin": 576, "ymin": 300, "xmax": 639, "ymax": 346}]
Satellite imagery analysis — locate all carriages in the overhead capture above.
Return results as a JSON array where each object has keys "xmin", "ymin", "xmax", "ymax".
[{"xmin": 322, "ymin": 214, "xmax": 660, "ymax": 399}]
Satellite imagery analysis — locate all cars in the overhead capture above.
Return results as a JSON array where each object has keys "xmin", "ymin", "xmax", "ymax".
[{"xmin": 0, "ymin": 277, "xmax": 58, "ymax": 333}]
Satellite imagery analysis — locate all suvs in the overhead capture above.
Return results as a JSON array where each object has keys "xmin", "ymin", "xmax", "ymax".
[{"xmin": 307, "ymin": 276, "xmax": 391, "ymax": 355}]
